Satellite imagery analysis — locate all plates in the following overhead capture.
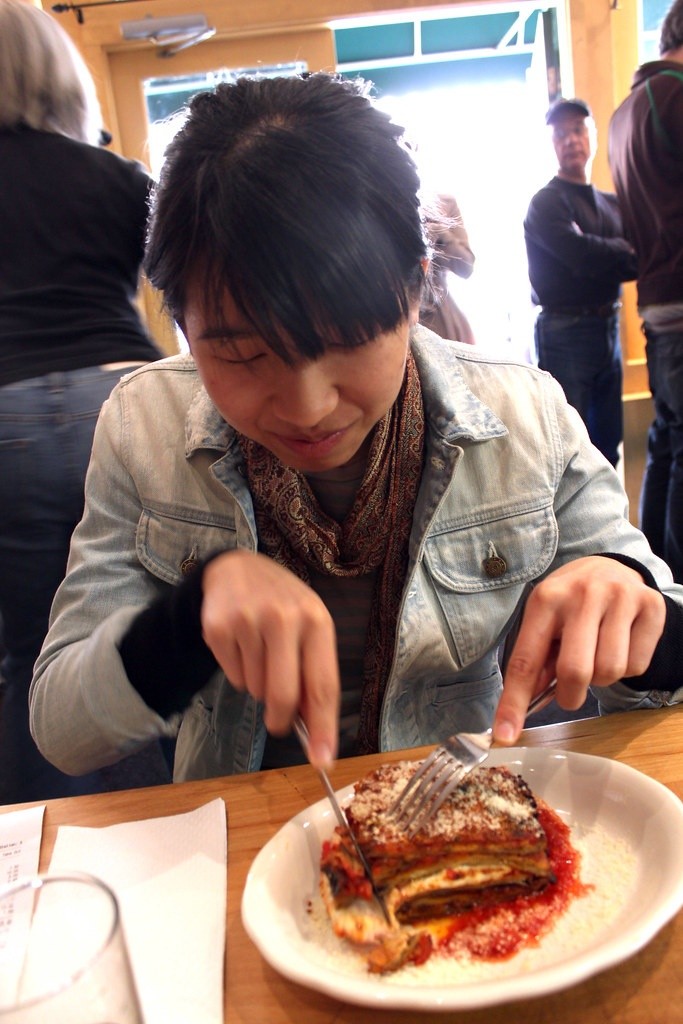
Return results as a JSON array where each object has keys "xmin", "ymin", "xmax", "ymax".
[{"xmin": 241, "ymin": 746, "xmax": 683, "ymax": 1011}]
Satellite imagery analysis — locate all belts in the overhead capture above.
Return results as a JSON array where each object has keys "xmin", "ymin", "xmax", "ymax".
[{"xmin": 542, "ymin": 298, "xmax": 623, "ymax": 318}]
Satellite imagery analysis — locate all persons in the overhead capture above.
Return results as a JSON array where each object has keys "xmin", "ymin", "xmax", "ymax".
[
  {"xmin": 522, "ymin": 99, "xmax": 639, "ymax": 471},
  {"xmin": 26, "ymin": 69, "xmax": 683, "ymax": 786},
  {"xmin": 0, "ymin": 0, "xmax": 173, "ymax": 806},
  {"xmin": 606, "ymin": 0, "xmax": 683, "ymax": 585}
]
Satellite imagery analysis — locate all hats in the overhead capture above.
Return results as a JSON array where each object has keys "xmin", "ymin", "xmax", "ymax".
[{"xmin": 544, "ymin": 98, "xmax": 590, "ymax": 125}]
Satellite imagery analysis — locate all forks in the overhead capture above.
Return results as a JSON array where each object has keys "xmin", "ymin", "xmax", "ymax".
[{"xmin": 385, "ymin": 678, "xmax": 558, "ymax": 839}]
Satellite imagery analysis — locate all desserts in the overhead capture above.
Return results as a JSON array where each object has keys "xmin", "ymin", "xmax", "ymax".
[{"xmin": 318, "ymin": 758, "xmax": 554, "ymax": 970}]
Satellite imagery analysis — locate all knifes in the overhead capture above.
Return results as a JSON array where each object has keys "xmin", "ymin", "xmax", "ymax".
[{"xmin": 292, "ymin": 714, "xmax": 392, "ymax": 930}]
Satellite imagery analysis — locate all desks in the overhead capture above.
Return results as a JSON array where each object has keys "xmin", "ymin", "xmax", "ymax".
[{"xmin": 1, "ymin": 703, "xmax": 683, "ymax": 1023}]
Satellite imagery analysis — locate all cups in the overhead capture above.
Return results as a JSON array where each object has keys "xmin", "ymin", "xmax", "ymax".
[{"xmin": 0, "ymin": 873, "xmax": 145, "ymax": 1024}]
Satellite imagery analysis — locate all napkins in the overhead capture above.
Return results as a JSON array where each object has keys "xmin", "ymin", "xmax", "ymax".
[{"xmin": 49, "ymin": 797, "xmax": 228, "ymax": 1024}]
